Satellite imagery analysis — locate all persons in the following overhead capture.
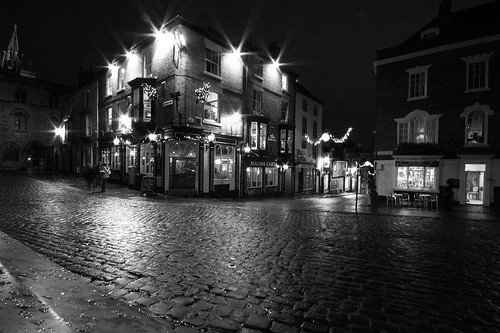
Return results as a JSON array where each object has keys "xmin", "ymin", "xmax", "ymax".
[
  {"xmin": 100, "ymin": 163, "xmax": 110, "ymax": 193},
  {"xmin": 91, "ymin": 164, "xmax": 96, "ymax": 188},
  {"xmin": 95, "ymin": 163, "xmax": 101, "ymax": 187},
  {"xmin": 437, "ymin": 177, "xmax": 463, "ymax": 240},
  {"xmin": 83, "ymin": 164, "xmax": 94, "ymax": 191}
]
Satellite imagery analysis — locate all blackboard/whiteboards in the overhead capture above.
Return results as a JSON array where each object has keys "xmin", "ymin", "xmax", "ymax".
[{"xmin": 141, "ymin": 176, "xmax": 157, "ymax": 192}]
